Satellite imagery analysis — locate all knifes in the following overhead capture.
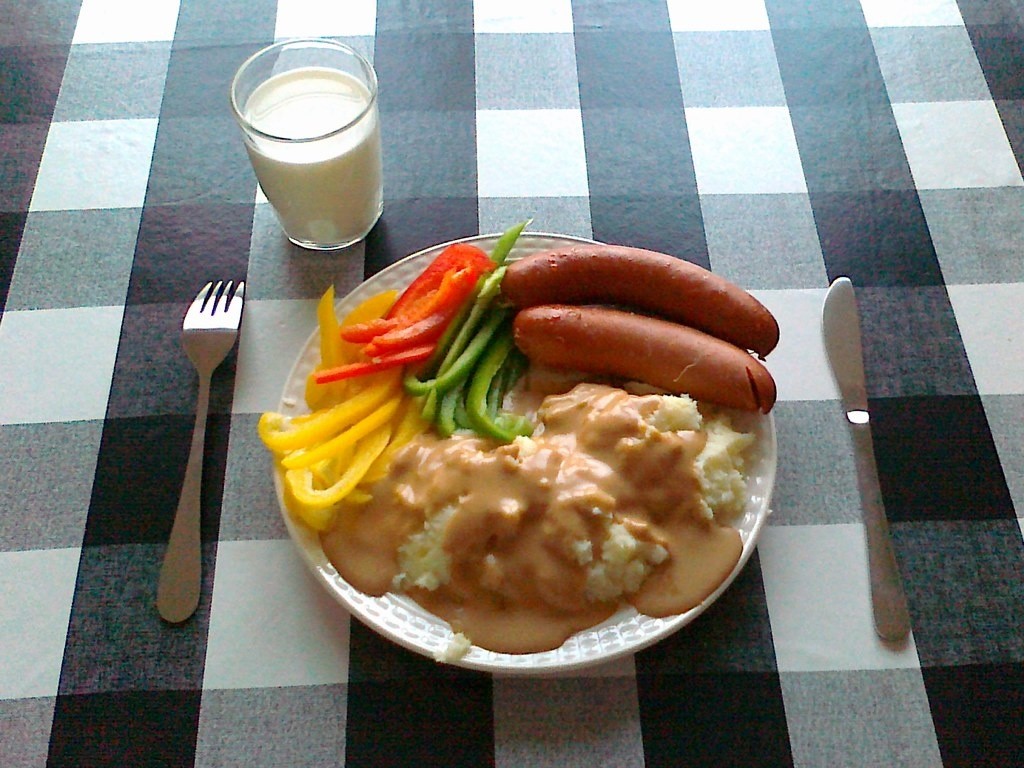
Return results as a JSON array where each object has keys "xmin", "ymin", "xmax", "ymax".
[{"xmin": 822, "ymin": 278, "xmax": 911, "ymax": 643}]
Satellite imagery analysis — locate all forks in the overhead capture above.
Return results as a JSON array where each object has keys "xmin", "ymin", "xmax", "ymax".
[{"xmin": 156, "ymin": 279, "xmax": 246, "ymax": 624}]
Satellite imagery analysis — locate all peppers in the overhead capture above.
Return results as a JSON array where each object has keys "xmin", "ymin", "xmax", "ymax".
[{"xmin": 258, "ymin": 219, "xmax": 529, "ymax": 529}]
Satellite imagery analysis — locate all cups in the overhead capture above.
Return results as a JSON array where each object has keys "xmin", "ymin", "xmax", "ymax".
[{"xmin": 228, "ymin": 38, "xmax": 385, "ymax": 253}]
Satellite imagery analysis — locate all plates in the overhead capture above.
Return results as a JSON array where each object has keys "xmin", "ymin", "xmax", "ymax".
[{"xmin": 271, "ymin": 229, "xmax": 778, "ymax": 677}]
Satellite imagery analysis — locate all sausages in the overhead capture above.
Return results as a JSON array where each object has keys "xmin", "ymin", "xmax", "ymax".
[{"xmin": 502, "ymin": 244, "xmax": 779, "ymax": 415}]
